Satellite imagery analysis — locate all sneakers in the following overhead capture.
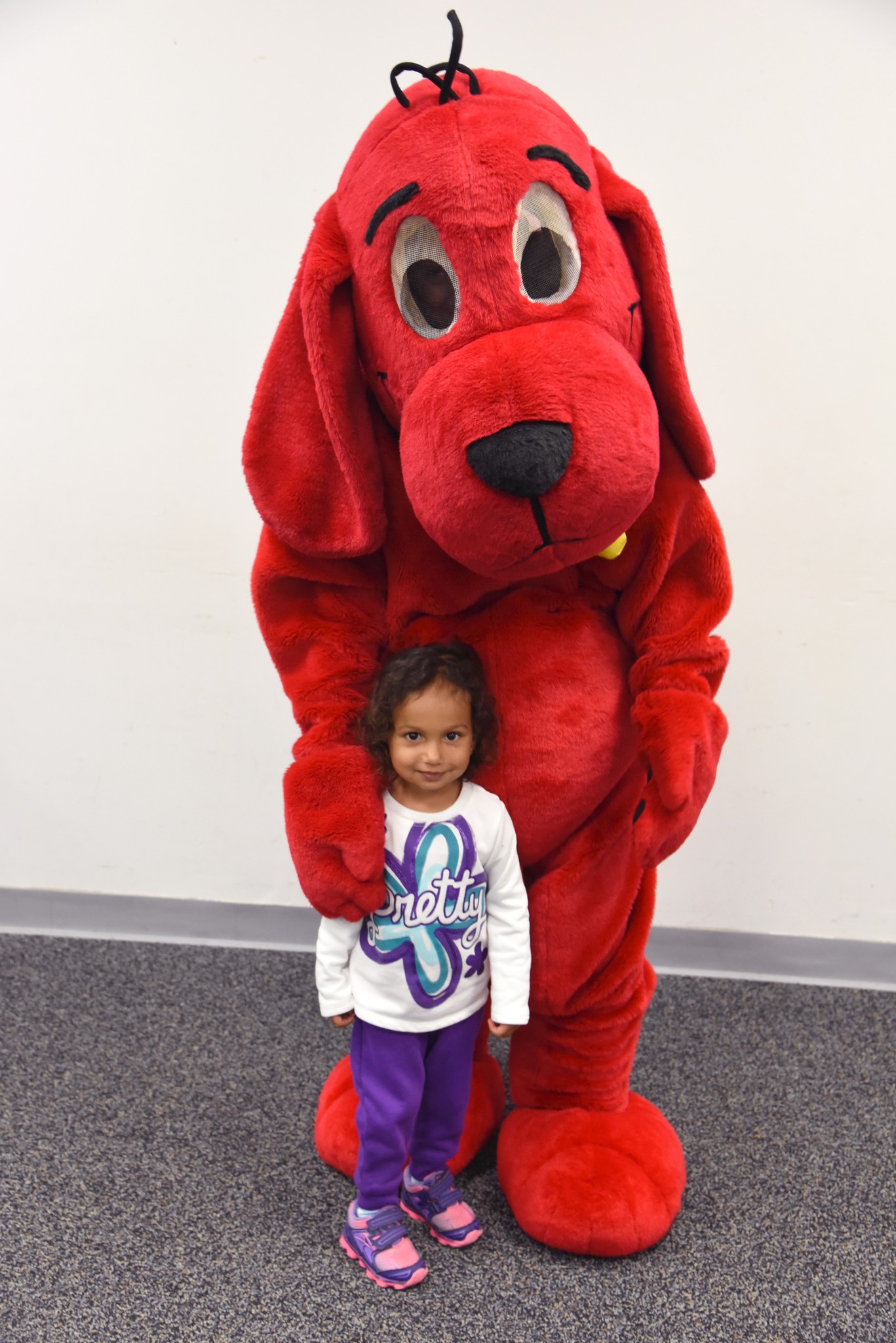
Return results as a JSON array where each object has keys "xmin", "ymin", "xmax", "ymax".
[
  {"xmin": 402, "ymin": 1165, "xmax": 481, "ymax": 1249},
  {"xmin": 340, "ymin": 1198, "xmax": 429, "ymax": 1289}
]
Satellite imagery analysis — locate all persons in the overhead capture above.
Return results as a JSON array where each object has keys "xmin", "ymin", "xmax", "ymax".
[{"xmin": 314, "ymin": 638, "xmax": 536, "ymax": 1287}]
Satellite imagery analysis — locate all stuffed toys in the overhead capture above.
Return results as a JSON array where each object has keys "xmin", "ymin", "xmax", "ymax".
[{"xmin": 241, "ymin": 7, "xmax": 736, "ymax": 1262}]
